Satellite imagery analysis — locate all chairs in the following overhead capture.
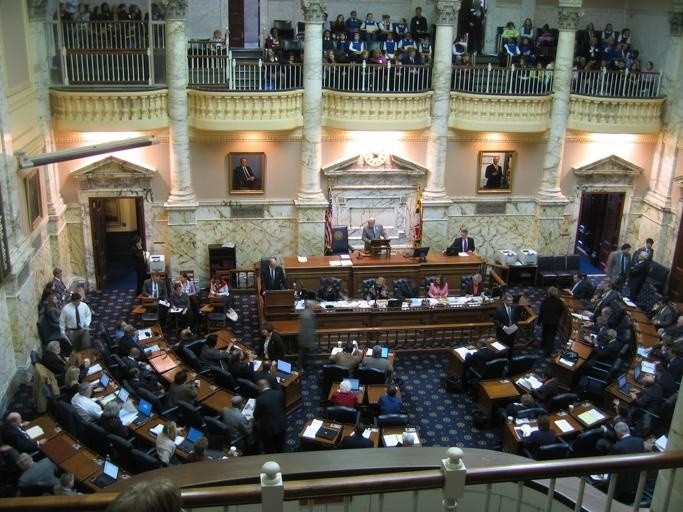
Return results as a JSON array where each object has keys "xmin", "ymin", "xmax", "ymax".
[
  {"xmin": 125, "ymin": 270, "xmax": 235, "ymax": 333},
  {"xmin": 491, "ymin": 250, "xmax": 576, "ymax": 289},
  {"xmin": 263, "ymin": 19, "xmax": 477, "ymax": 66},
  {"xmin": 445, "ymin": 279, "xmax": 681, "ymax": 461},
  {"xmin": 3, "ymin": 323, "xmax": 304, "ymax": 499},
  {"xmin": 495, "ymin": 27, "xmax": 641, "ymax": 73},
  {"xmin": 53, "ymin": 5, "xmax": 164, "ymax": 48},
  {"xmin": 299, "ymin": 343, "xmax": 423, "ymax": 452}
]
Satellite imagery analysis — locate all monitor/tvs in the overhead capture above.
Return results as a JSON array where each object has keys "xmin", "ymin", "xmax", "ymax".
[
  {"xmin": 413, "ymin": 246, "xmax": 430, "ymax": 262},
  {"xmin": 491, "ymin": 285, "xmax": 506, "ymax": 299},
  {"xmin": 388, "ymin": 299, "xmax": 402, "ymax": 311}
]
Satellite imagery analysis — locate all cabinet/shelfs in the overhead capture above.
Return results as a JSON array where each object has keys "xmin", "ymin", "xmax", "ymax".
[{"xmin": 209, "ymin": 243, "xmax": 237, "ymax": 289}]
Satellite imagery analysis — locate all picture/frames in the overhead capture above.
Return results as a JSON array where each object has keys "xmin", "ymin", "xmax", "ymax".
[
  {"xmin": 476, "ymin": 148, "xmax": 516, "ymax": 194},
  {"xmin": 224, "ymin": 148, "xmax": 268, "ymax": 197},
  {"xmin": 23, "ymin": 167, "xmax": 44, "ymax": 233}
]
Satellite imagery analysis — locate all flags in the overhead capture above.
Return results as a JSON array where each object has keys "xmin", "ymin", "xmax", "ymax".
[
  {"xmin": 413, "ymin": 188, "xmax": 422, "ymax": 248},
  {"xmin": 325, "ymin": 194, "xmax": 334, "ymax": 253}
]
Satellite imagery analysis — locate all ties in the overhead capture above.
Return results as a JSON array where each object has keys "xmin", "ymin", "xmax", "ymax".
[
  {"xmin": 270, "ymin": 269, "xmax": 274, "ymax": 281},
  {"xmin": 153, "ymin": 283, "xmax": 156, "ymax": 297},
  {"xmin": 244, "ymin": 167, "xmax": 251, "ymax": 180},
  {"xmin": 464, "ymin": 240, "xmax": 466, "ymax": 252},
  {"xmin": 75, "ymin": 306, "xmax": 80, "ymax": 328}
]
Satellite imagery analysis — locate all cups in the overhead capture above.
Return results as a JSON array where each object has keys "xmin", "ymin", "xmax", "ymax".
[
  {"xmin": 507, "ymin": 416, "xmax": 513, "ymax": 425},
  {"xmin": 337, "ymin": 340, "xmax": 342, "ymax": 348},
  {"xmin": 568, "ymin": 405, "xmax": 574, "ymax": 413},
  {"xmin": 566, "ymin": 340, "xmax": 572, "ymax": 349},
  {"xmin": 194, "ymin": 380, "xmax": 200, "ymax": 388}
]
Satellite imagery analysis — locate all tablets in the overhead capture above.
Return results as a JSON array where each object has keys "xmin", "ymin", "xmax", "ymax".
[{"xmin": 446, "ymin": 248, "xmax": 458, "ymax": 255}]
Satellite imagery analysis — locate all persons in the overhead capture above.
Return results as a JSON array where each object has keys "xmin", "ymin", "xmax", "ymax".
[
  {"xmin": 262, "ymin": 7, "xmax": 484, "ymax": 90},
  {"xmin": 234, "ymin": 158, "xmax": 257, "ymax": 189},
  {"xmin": 208, "ymin": 29, "xmax": 226, "ymax": 65},
  {"xmin": 51, "ymin": 0, "xmax": 167, "ymax": 52},
  {"xmin": 500, "ymin": 17, "xmax": 655, "ymax": 97},
  {"xmin": 486, "ymin": 155, "xmax": 503, "ymax": 187}
]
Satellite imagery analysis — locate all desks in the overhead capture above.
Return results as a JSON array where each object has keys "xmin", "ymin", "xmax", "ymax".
[{"xmin": 254, "ymin": 250, "xmax": 531, "ymax": 342}]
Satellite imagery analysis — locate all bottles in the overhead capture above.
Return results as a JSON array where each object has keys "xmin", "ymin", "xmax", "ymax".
[
  {"xmin": 105, "ymin": 454, "xmax": 110, "ymax": 462},
  {"xmin": 480, "ymin": 292, "xmax": 484, "ymax": 303}
]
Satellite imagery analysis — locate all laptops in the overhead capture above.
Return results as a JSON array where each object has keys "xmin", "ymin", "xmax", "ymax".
[
  {"xmin": 92, "ymin": 460, "xmax": 119, "ymax": 488},
  {"xmin": 381, "ymin": 345, "xmax": 389, "ymax": 360},
  {"xmin": 617, "ymin": 374, "xmax": 631, "ymax": 396},
  {"xmin": 315, "ymin": 427, "xmax": 337, "ymax": 440},
  {"xmin": 343, "ymin": 377, "xmax": 360, "ymax": 393},
  {"xmin": 178, "ymin": 426, "xmax": 206, "ymax": 454},
  {"xmin": 111, "ymin": 386, "xmax": 129, "ymax": 409},
  {"xmin": 634, "ymin": 365, "xmax": 644, "ymax": 385},
  {"xmin": 133, "ymin": 398, "xmax": 153, "ymax": 425},
  {"xmin": 276, "ymin": 359, "xmax": 292, "ymax": 382},
  {"xmin": 94, "ymin": 372, "xmax": 110, "ymax": 393}
]
